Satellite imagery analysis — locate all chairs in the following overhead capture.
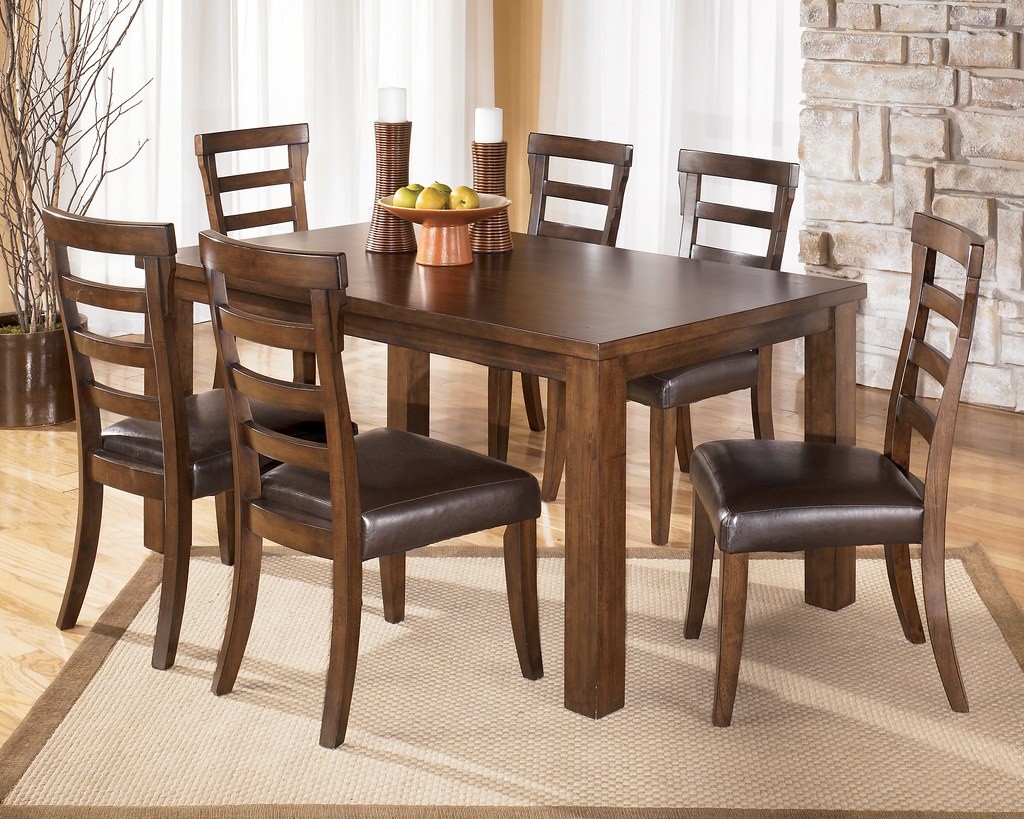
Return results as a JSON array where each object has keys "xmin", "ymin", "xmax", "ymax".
[
  {"xmin": 489, "ymin": 133, "xmax": 630, "ymax": 459},
  {"xmin": 200, "ymin": 226, "xmax": 540, "ymax": 752},
  {"xmin": 542, "ymin": 151, "xmax": 793, "ymax": 544},
  {"xmin": 684, "ymin": 214, "xmax": 986, "ymax": 728},
  {"xmin": 195, "ymin": 123, "xmax": 322, "ymax": 390}
]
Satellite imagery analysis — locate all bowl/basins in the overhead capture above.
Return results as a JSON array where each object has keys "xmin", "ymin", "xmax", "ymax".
[{"xmin": 378, "ymin": 193, "xmax": 513, "ymax": 267}]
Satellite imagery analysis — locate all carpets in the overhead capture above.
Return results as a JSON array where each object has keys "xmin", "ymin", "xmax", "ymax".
[
  {"xmin": 44, "ymin": 207, "xmax": 360, "ymax": 673},
  {"xmin": 0, "ymin": 538, "xmax": 1024, "ymax": 819}
]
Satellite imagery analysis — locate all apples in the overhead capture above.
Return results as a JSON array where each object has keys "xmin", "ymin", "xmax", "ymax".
[{"xmin": 393, "ymin": 180, "xmax": 480, "ymax": 210}]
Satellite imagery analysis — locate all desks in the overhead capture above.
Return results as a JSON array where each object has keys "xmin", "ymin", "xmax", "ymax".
[{"xmin": 132, "ymin": 216, "xmax": 870, "ymax": 720}]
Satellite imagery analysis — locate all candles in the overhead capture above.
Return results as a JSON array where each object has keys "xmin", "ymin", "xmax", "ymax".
[
  {"xmin": 378, "ymin": 87, "xmax": 408, "ymax": 123},
  {"xmin": 474, "ymin": 107, "xmax": 503, "ymax": 143}
]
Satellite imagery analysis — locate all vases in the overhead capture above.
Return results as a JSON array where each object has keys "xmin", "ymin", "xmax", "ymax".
[{"xmin": 0, "ymin": 312, "xmax": 97, "ymax": 431}]
